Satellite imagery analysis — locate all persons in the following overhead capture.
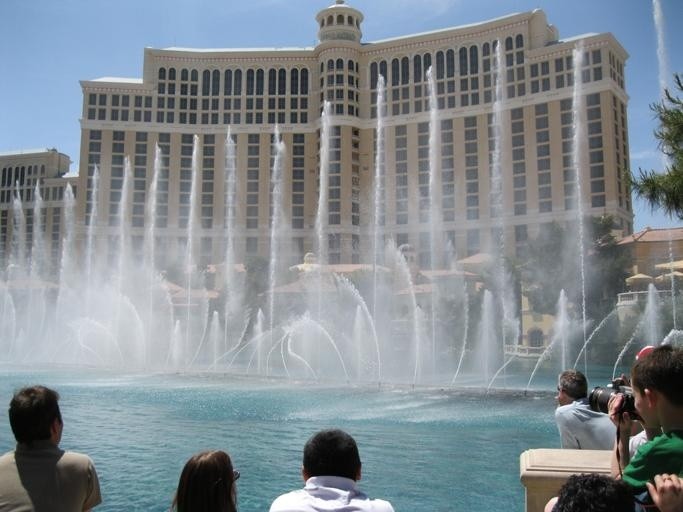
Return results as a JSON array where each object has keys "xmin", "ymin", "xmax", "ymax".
[
  {"xmin": 0, "ymin": 385, "xmax": 103, "ymax": 511},
  {"xmin": 169, "ymin": 451, "xmax": 240, "ymax": 512},
  {"xmin": 542, "ymin": 346, "xmax": 683, "ymax": 512},
  {"xmin": 267, "ymin": 430, "xmax": 396, "ymax": 512}
]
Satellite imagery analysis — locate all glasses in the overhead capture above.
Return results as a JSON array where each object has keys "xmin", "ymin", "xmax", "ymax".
[{"xmin": 232, "ymin": 469, "xmax": 241, "ymax": 483}]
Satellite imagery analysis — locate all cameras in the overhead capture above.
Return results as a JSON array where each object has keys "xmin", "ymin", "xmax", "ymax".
[
  {"xmin": 588, "ymin": 378, "xmax": 643, "ymax": 421},
  {"xmin": 635, "ymin": 481, "xmax": 660, "ymax": 512}
]
